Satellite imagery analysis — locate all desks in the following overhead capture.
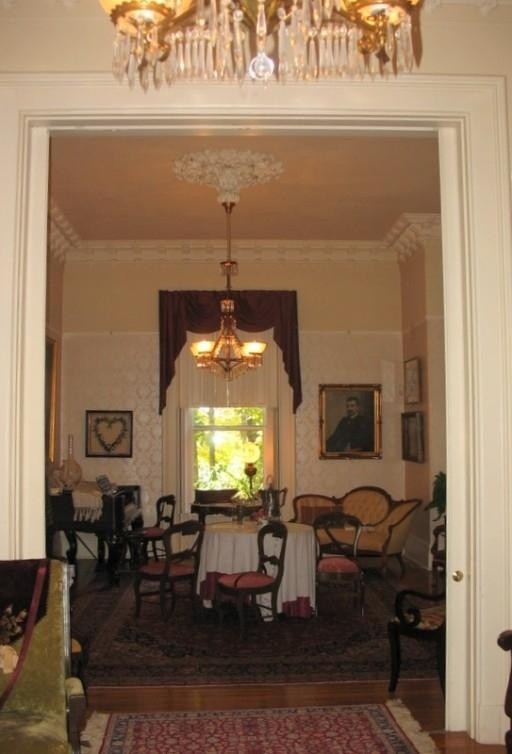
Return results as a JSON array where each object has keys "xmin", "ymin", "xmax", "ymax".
[
  {"xmin": 46, "ymin": 481, "xmax": 144, "ymax": 575},
  {"xmin": 196, "ymin": 520, "xmax": 318, "ymax": 618},
  {"xmin": 191, "ymin": 502, "xmax": 271, "ymax": 532}
]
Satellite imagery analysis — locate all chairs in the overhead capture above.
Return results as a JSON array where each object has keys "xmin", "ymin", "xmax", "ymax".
[
  {"xmin": 139, "ymin": 494, "xmax": 175, "ymax": 566},
  {"xmin": 0, "ymin": 558, "xmax": 74, "ymax": 754},
  {"xmin": 213, "ymin": 522, "xmax": 288, "ymax": 639},
  {"xmin": 315, "ymin": 514, "xmax": 366, "ymax": 608},
  {"xmin": 132, "ymin": 521, "xmax": 205, "ymax": 622},
  {"xmin": 432, "ymin": 524, "xmax": 447, "ymax": 594},
  {"xmin": 386, "ymin": 524, "xmax": 447, "ymax": 699}
]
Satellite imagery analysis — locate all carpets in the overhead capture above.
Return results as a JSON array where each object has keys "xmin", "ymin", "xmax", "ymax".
[
  {"xmin": 70, "ymin": 556, "xmax": 446, "ymax": 688},
  {"xmin": 80, "ymin": 699, "xmax": 443, "ymax": 754}
]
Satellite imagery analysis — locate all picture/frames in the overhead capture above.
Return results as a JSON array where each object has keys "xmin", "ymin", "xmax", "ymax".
[
  {"xmin": 317, "ymin": 382, "xmax": 383, "ymax": 462},
  {"xmin": 85, "ymin": 409, "xmax": 134, "ymax": 459},
  {"xmin": 400, "ymin": 410, "xmax": 426, "ymax": 464},
  {"xmin": 401, "ymin": 356, "xmax": 422, "ymax": 405}
]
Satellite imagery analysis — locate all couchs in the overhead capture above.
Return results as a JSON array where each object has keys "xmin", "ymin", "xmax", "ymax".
[{"xmin": 288, "ymin": 485, "xmax": 421, "ymax": 573}]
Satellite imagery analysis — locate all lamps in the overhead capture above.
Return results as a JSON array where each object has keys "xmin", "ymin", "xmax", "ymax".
[
  {"xmin": 97, "ymin": 0, "xmax": 418, "ymax": 97},
  {"xmin": 170, "ymin": 145, "xmax": 286, "ymax": 379}
]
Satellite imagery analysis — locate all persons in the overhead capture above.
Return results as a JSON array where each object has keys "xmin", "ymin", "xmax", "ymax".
[{"xmin": 325, "ymin": 396, "xmax": 374, "ymax": 453}]
